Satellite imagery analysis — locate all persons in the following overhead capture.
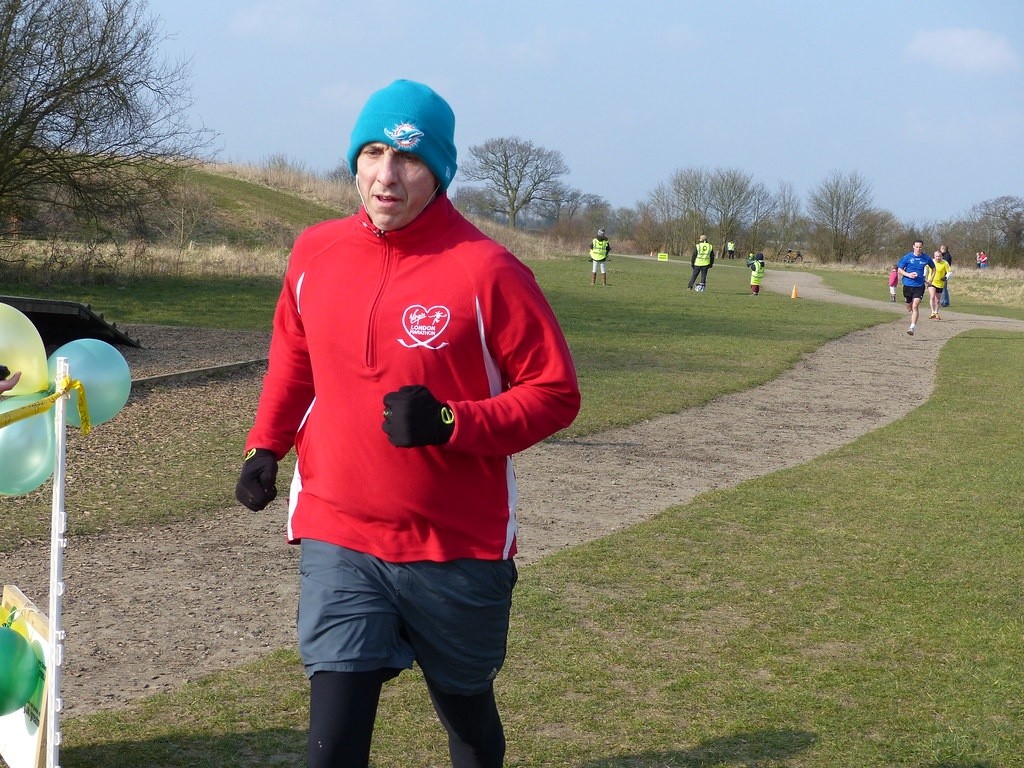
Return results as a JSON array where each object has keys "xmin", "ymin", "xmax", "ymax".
[
  {"xmin": 750, "ymin": 252, "xmax": 765, "ymax": 296},
  {"xmin": 898, "ymin": 240, "xmax": 936, "ymax": 335},
  {"xmin": 888, "ymin": 265, "xmax": 898, "ymax": 302},
  {"xmin": 974, "ymin": 251, "xmax": 988, "ymax": 269},
  {"xmin": 236, "ymin": 79, "xmax": 579, "ymax": 768},
  {"xmin": 687, "ymin": 234, "xmax": 714, "ymax": 291},
  {"xmin": 924, "ymin": 249, "xmax": 951, "ymax": 321},
  {"xmin": 727, "ymin": 240, "xmax": 735, "ymax": 259},
  {"xmin": 939, "ymin": 245, "xmax": 952, "ymax": 307},
  {"xmin": 589, "ymin": 227, "xmax": 610, "ymax": 286}
]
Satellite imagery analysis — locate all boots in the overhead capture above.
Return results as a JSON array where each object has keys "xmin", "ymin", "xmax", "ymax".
[
  {"xmin": 591, "ymin": 273, "xmax": 596, "ymax": 284},
  {"xmin": 892, "ymin": 295, "xmax": 895, "ymax": 302},
  {"xmin": 601, "ymin": 273, "xmax": 606, "ymax": 285}
]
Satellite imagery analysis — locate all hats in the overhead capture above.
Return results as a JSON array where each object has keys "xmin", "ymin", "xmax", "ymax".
[
  {"xmin": 756, "ymin": 253, "xmax": 763, "ymax": 260},
  {"xmin": 346, "ymin": 78, "xmax": 458, "ymax": 195},
  {"xmin": 599, "ymin": 228, "xmax": 605, "ymax": 233}
]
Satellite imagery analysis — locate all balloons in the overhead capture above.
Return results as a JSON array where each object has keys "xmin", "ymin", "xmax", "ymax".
[
  {"xmin": 0, "ymin": 627, "xmax": 39, "ymax": 717},
  {"xmin": 47, "ymin": 339, "xmax": 131, "ymax": 428},
  {"xmin": 0, "ymin": 303, "xmax": 49, "ymax": 396},
  {"xmin": 0, "ymin": 394, "xmax": 56, "ymax": 496}
]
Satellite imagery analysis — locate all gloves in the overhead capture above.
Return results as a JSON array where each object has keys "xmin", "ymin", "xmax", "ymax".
[
  {"xmin": 381, "ymin": 385, "xmax": 455, "ymax": 448},
  {"xmin": 235, "ymin": 447, "xmax": 278, "ymax": 512}
]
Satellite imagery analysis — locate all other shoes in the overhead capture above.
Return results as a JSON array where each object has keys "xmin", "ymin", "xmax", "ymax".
[
  {"xmin": 929, "ymin": 313, "xmax": 935, "ymax": 319},
  {"xmin": 935, "ymin": 313, "xmax": 940, "ymax": 320},
  {"xmin": 687, "ymin": 287, "xmax": 691, "ymax": 291},
  {"xmin": 907, "ymin": 328, "xmax": 914, "ymax": 335},
  {"xmin": 753, "ymin": 292, "xmax": 758, "ymax": 295}
]
